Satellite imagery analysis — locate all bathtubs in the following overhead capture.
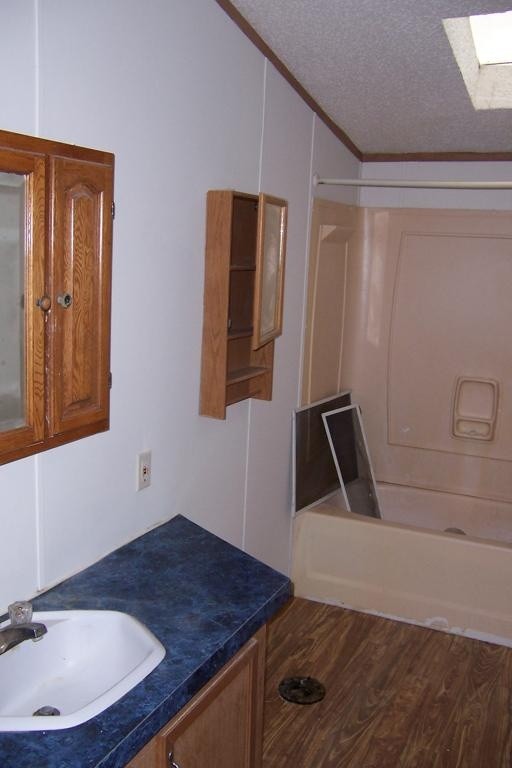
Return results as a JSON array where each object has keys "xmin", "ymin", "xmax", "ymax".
[{"xmin": 304, "ymin": 478, "xmax": 511, "ymax": 642}]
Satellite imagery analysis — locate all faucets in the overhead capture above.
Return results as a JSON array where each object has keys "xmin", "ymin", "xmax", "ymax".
[{"xmin": 1, "ymin": 602, "xmax": 46, "ymax": 655}]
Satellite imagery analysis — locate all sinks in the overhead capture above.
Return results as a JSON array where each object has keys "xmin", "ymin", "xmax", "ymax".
[{"xmin": 1, "ymin": 609, "xmax": 166, "ymax": 731}]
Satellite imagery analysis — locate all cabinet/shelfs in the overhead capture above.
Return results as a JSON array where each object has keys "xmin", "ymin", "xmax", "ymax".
[
  {"xmin": 1, "ymin": 131, "xmax": 115, "ymax": 467},
  {"xmin": 160, "ymin": 625, "xmax": 266, "ymax": 768},
  {"xmin": 200, "ymin": 191, "xmax": 289, "ymax": 420}
]
